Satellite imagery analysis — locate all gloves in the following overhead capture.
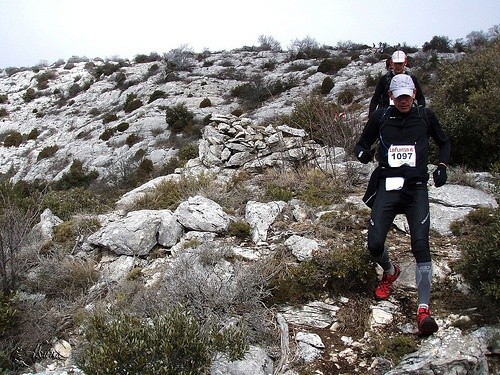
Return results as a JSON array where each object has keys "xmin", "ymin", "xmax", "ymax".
[
  {"xmin": 433, "ymin": 164, "xmax": 447, "ymax": 188},
  {"xmin": 355, "ymin": 147, "xmax": 373, "ymax": 165}
]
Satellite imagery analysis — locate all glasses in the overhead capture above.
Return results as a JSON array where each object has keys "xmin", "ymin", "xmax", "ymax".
[{"xmin": 394, "ymin": 62, "xmax": 403, "ymax": 65}]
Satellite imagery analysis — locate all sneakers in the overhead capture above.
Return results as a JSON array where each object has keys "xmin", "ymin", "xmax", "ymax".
[
  {"xmin": 375, "ymin": 264, "xmax": 400, "ymax": 299},
  {"xmin": 415, "ymin": 307, "xmax": 439, "ymax": 339}
]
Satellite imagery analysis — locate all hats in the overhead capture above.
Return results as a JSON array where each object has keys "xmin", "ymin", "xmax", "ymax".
[
  {"xmin": 390, "ymin": 74, "xmax": 415, "ymax": 98},
  {"xmin": 392, "ymin": 50, "xmax": 407, "ymax": 63}
]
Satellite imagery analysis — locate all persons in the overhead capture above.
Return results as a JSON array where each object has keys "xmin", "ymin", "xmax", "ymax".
[
  {"xmin": 368, "ymin": 50, "xmax": 426, "ymax": 118},
  {"xmin": 386, "ymin": 57, "xmax": 393, "ymax": 72},
  {"xmin": 354, "ymin": 74, "xmax": 452, "ymax": 334}
]
